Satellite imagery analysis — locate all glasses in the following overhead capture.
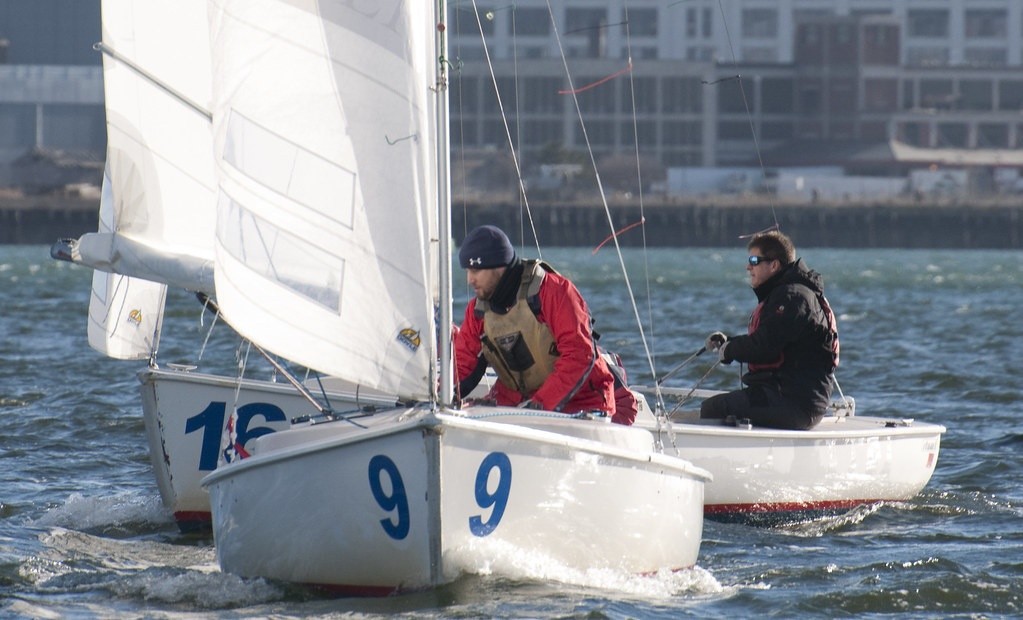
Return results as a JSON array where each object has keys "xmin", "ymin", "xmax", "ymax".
[{"xmin": 748, "ymin": 255, "xmax": 775, "ymax": 266}]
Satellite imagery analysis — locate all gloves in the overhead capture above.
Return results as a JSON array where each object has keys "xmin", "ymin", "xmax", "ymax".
[
  {"xmin": 706, "ymin": 332, "xmax": 726, "ymax": 350},
  {"xmin": 718, "ymin": 341, "xmax": 735, "ymax": 364}
]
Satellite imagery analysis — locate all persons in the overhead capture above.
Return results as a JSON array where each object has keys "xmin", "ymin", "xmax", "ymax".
[
  {"xmin": 451, "ymin": 224, "xmax": 641, "ymax": 428},
  {"xmin": 700, "ymin": 231, "xmax": 838, "ymax": 431}
]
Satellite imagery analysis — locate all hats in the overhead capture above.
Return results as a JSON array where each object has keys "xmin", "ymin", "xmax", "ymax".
[{"xmin": 459, "ymin": 224, "xmax": 514, "ymax": 268}]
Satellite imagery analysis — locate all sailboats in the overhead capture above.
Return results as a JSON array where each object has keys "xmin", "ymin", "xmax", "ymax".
[
  {"xmin": 93, "ymin": 1, "xmax": 709, "ymax": 596},
  {"xmin": 138, "ymin": 0, "xmax": 950, "ymax": 528}
]
